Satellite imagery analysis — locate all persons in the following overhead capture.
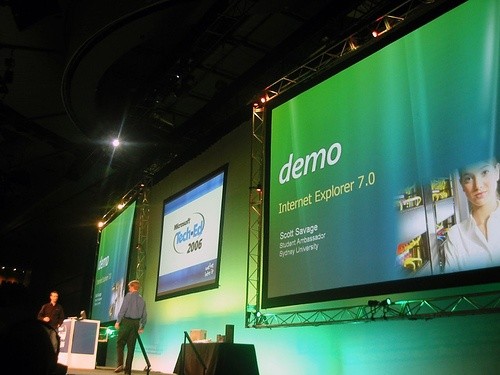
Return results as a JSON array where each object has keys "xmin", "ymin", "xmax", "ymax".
[
  {"xmin": 444, "ymin": 159, "xmax": 500, "ymax": 271},
  {"xmin": 38, "ymin": 291, "xmax": 64, "ymax": 357},
  {"xmin": 109, "ymin": 280, "xmax": 124, "ymax": 319},
  {"xmin": 114, "ymin": 280, "xmax": 147, "ymax": 375}
]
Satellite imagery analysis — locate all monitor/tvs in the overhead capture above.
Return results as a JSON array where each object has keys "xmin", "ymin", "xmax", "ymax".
[{"xmin": 80, "ymin": 310, "xmax": 86, "ymax": 319}]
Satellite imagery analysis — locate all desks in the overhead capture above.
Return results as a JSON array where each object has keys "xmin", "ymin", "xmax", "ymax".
[{"xmin": 171, "ymin": 342, "xmax": 260, "ymax": 375}]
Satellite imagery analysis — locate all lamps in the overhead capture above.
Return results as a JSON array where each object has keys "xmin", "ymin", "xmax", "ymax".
[{"xmin": 367, "ymin": 297, "xmax": 392, "ymax": 307}]
